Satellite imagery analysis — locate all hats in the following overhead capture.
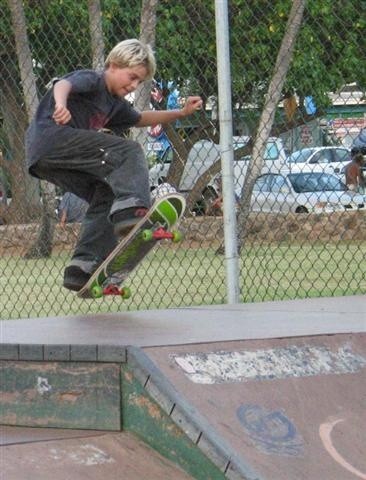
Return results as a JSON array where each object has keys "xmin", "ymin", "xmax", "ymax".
[
  {"xmin": 287, "ymin": 146, "xmax": 354, "ymax": 183},
  {"xmin": 235, "ymin": 171, "xmax": 366, "ymax": 212}
]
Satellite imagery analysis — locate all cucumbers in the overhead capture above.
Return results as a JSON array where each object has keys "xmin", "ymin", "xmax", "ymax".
[{"xmin": 76, "ymin": 191, "xmax": 188, "ymax": 300}]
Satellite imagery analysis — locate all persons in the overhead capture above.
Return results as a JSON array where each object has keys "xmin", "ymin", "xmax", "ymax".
[
  {"xmin": 59, "ymin": 189, "xmax": 89, "ymax": 223},
  {"xmin": 146, "ymin": 76, "xmax": 179, "ymax": 182},
  {"xmin": 22, "ymin": 37, "xmax": 205, "ymax": 294}
]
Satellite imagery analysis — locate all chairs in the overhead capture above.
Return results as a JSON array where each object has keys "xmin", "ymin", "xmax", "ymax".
[
  {"xmin": 63, "ymin": 261, "xmax": 96, "ymax": 291},
  {"xmin": 112, "ymin": 206, "xmax": 148, "ymax": 235}
]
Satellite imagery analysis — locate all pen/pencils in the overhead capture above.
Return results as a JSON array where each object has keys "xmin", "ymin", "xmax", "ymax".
[{"xmin": 148, "ymin": 136, "xmax": 286, "ymax": 207}]
